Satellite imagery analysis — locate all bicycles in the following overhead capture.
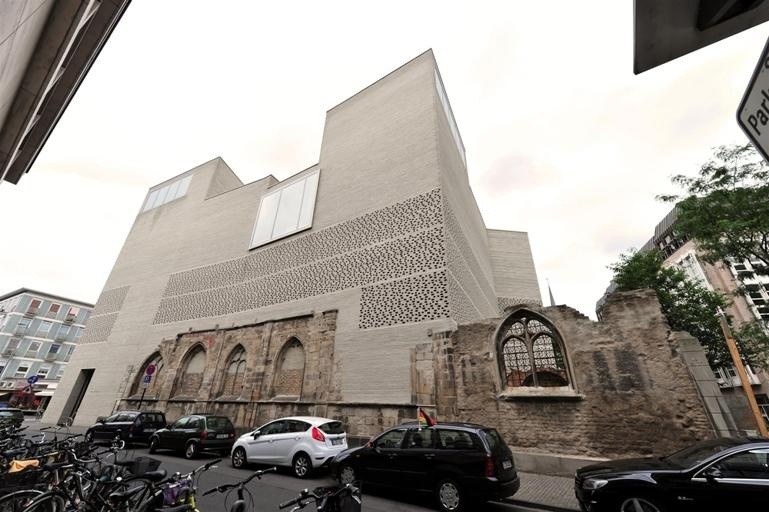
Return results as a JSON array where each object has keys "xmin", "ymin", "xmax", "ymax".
[
  {"xmin": 278, "ymin": 477, "xmax": 363, "ymax": 512},
  {"xmin": 202, "ymin": 467, "xmax": 278, "ymax": 512},
  {"xmin": 0, "ymin": 426, "xmax": 223, "ymax": 512}
]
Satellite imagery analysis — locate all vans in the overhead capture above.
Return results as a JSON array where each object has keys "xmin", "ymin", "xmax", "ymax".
[{"xmin": 329, "ymin": 422, "xmax": 518, "ymax": 512}]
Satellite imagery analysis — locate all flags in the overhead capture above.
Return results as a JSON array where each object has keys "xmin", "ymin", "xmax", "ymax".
[{"xmin": 417, "ymin": 407, "xmax": 436, "ymax": 426}]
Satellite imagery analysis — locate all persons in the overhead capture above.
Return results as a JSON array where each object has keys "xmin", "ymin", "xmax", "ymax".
[
  {"xmin": 445, "ymin": 437, "xmax": 456, "ymax": 450},
  {"xmin": 288, "ymin": 423, "xmax": 297, "ymax": 432}
]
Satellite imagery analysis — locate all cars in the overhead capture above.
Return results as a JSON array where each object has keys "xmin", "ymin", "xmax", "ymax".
[
  {"xmin": 83, "ymin": 411, "xmax": 162, "ymax": 447},
  {"xmin": 147, "ymin": 413, "xmax": 235, "ymax": 460},
  {"xmin": 0, "ymin": 407, "xmax": 24, "ymax": 428},
  {"xmin": 573, "ymin": 438, "xmax": 769, "ymax": 511},
  {"xmin": 231, "ymin": 416, "xmax": 349, "ymax": 478}
]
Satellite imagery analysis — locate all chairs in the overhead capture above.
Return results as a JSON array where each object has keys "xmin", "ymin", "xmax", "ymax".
[{"xmin": 444, "ymin": 436, "xmax": 454, "ymax": 449}]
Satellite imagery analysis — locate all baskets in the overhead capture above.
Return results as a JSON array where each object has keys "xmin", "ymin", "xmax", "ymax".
[
  {"xmin": 128, "ymin": 457, "xmax": 162, "ymax": 475},
  {"xmin": 314, "ymin": 482, "xmax": 362, "ymax": 512}
]
